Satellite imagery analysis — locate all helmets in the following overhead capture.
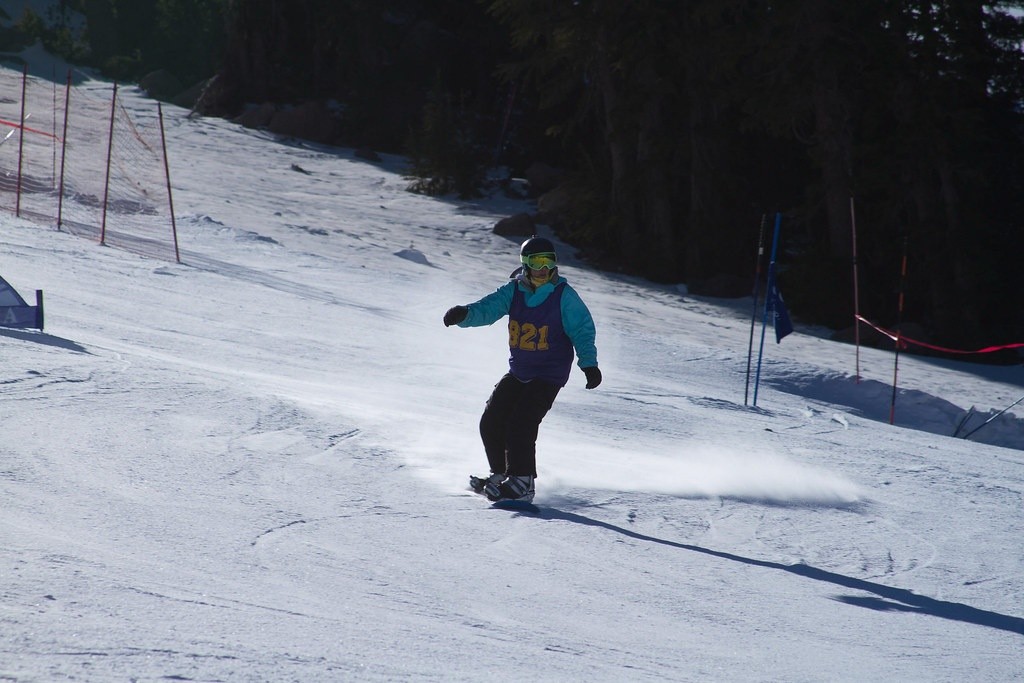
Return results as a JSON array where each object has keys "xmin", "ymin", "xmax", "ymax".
[{"xmin": 522, "ymin": 236, "xmax": 557, "ymax": 275}]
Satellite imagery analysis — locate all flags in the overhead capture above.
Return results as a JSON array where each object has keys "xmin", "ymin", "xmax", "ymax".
[{"xmin": 756, "ymin": 212, "xmax": 793, "ymax": 344}]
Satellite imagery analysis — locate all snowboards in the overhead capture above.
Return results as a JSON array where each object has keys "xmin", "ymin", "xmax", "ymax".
[{"xmin": 490, "ymin": 499, "xmax": 542, "ymax": 515}]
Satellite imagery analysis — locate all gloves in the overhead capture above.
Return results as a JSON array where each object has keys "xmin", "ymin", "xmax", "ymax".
[
  {"xmin": 585, "ymin": 367, "xmax": 602, "ymax": 389},
  {"xmin": 444, "ymin": 305, "xmax": 468, "ymax": 327}
]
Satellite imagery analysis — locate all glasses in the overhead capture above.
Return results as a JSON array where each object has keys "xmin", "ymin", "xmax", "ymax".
[{"xmin": 527, "ymin": 252, "xmax": 557, "ymax": 270}]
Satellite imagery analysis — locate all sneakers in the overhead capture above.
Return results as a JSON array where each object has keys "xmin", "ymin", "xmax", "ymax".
[
  {"xmin": 469, "ymin": 472, "xmax": 507, "ymax": 494},
  {"xmin": 484, "ymin": 474, "xmax": 535, "ymax": 504}
]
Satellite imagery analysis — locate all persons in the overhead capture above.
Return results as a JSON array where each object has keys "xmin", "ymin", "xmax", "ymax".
[{"xmin": 443, "ymin": 237, "xmax": 602, "ymax": 500}]
